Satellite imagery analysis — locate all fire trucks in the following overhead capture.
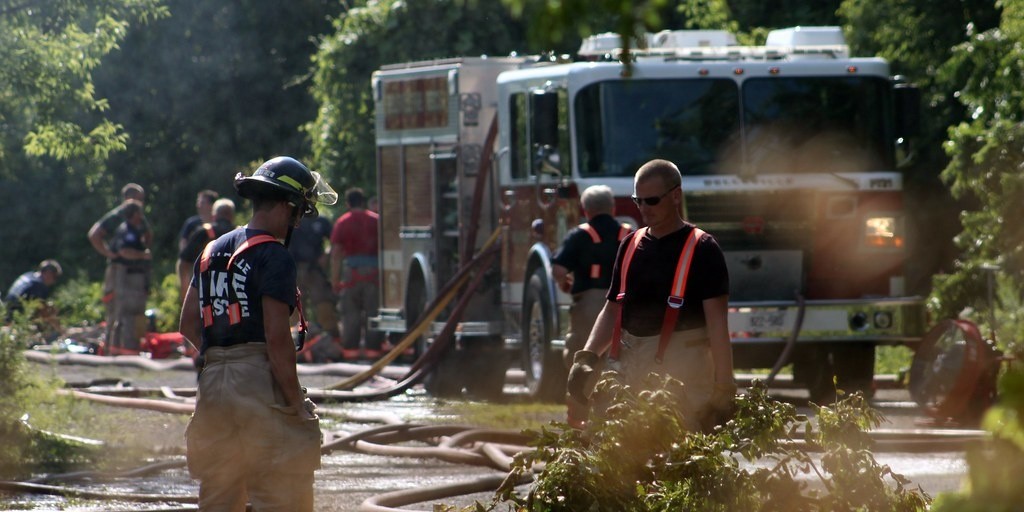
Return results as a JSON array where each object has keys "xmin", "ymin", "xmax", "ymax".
[{"xmin": 371, "ymin": 26, "xmax": 936, "ymax": 411}]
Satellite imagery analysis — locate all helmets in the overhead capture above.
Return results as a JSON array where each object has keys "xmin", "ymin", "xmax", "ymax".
[{"xmin": 233, "ymin": 155, "xmax": 339, "ymax": 219}]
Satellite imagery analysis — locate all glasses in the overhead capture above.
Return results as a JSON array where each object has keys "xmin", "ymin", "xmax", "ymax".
[{"xmin": 630, "ymin": 183, "xmax": 681, "ymax": 206}]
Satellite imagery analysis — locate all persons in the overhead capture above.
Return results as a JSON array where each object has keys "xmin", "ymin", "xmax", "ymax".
[
  {"xmin": 87, "ymin": 183, "xmax": 154, "ymax": 357},
  {"xmin": 288, "ymin": 188, "xmax": 384, "ymax": 365},
  {"xmin": 176, "ymin": 191, "xmax": 235, "ymax": 358},
  {"xmin": 5, "ymin": 258, "xmax": 62, "ymax": 326},
  {"xmin": 567, "ymin": 159, "xmax": 736, "ymax": 446},
  {"xmin": 179, "ymin": 156, "xmax": 321, "ymax": 512},
  {"xmin": 550, "ymin": 185, "xmax": 635, "ymax": 434}
]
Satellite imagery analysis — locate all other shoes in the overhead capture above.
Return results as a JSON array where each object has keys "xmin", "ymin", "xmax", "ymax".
[
  {"xmin": 96, "ymin": 342, "xmax": 141, "ymax": 356},
  {"xmin": 341, "ymin": 346, "xmax": 359, "ymax": 362},
  {"xmin": 565, "ymin": 415, "xmax": 587, "ymax": 431},
  {"xmin": 365, "ymin": 349, "xmax": 383, "ymax": 362}
]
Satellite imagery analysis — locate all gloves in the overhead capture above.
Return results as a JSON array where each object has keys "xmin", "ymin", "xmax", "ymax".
[
  {"xmin": 567, "ymin": 349, "xmax": 600, "ymax": 406},
  {"xmin": 698, "ymin": 381, "xmax": 738, "ymax": 434}
]
